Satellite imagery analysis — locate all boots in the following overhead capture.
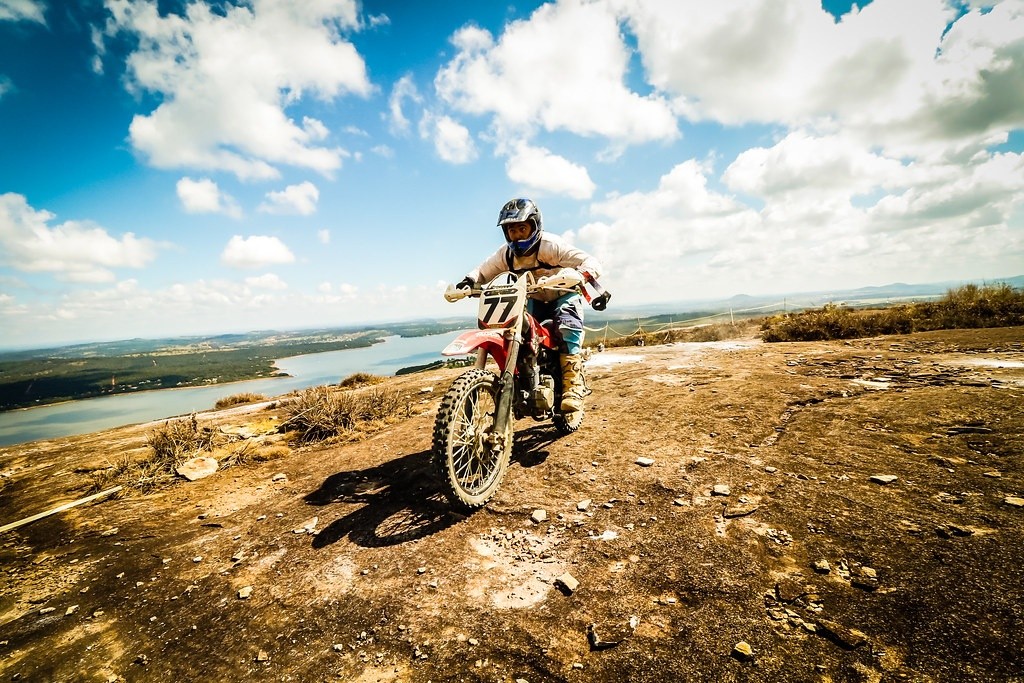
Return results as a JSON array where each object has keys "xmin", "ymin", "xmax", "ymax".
[{"xmin": 560, "ymin": 355, "xmax": 584, "ymax": 412}]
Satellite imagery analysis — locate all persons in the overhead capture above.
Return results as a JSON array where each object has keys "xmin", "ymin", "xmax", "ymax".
[{"xmin": 456, "ymin": 198, "xmax": 603, "ymax": 412}]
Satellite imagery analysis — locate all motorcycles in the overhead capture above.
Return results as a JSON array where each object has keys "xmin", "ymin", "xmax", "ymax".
[{"xmin": 430, "ymin": 270, "xmax": 593, "ymax": 512}]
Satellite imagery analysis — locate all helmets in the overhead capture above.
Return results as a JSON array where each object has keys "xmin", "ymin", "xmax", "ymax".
[{"xmin": 497, "ymin": 198, "xmax": 542, "ymax": 257}]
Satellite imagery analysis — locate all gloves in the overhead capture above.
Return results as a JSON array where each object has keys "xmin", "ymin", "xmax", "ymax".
[{"xmin": 456, "ymin": 277, "xmax": 474, "ymax": 298}]
